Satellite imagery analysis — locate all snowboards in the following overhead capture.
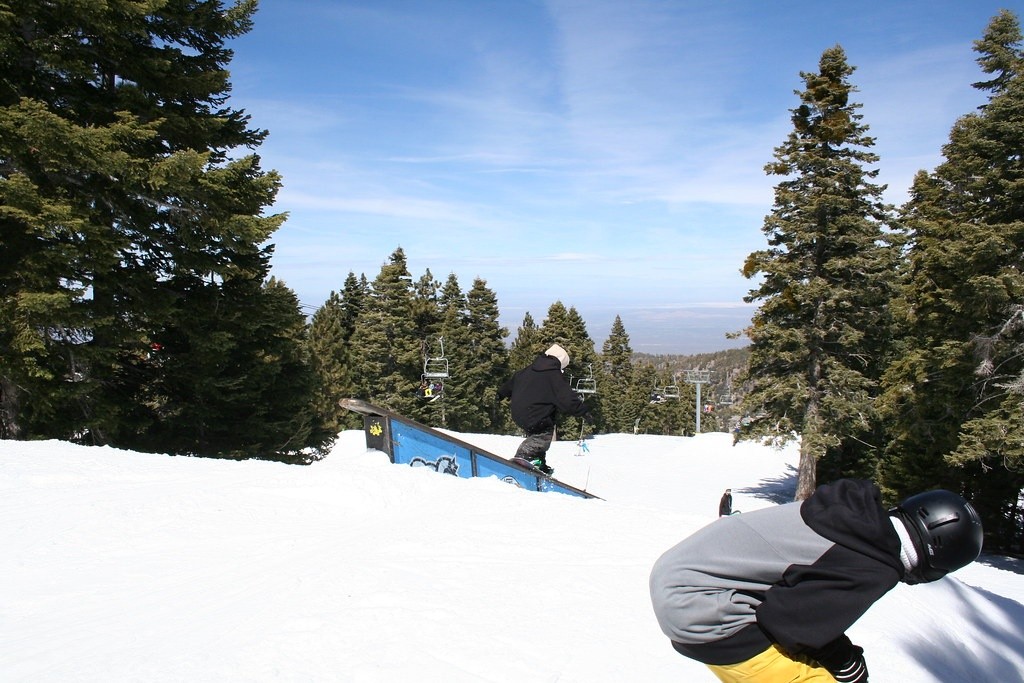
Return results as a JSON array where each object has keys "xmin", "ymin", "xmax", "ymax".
[{"xmin": 508, "ymin": 457, "xmax": 554, "ymax": 477}]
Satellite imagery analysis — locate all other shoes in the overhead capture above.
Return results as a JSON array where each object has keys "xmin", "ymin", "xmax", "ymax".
[
  {"xmin": 515, "ymin": 450, "xmax": 542, "ymax": 469},
  {"xmin": 538, "ymin": 452, "xmax": 554, "ymax": 477}
]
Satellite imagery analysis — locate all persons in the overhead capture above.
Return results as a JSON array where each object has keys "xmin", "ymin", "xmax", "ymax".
[
  {"xmin": 649, "ymin": 476, "xmax": 985, "ymax": 683},
  {"xmin": 719, "ymin": 488, "xmax": 733, "ymax": 518},
  {"xmin": 575, "ymin": 437, "xmax": 591, "ymax": 455},
  {"xmin": 418, "ymin": 379, "xmax": 443, "ymax": 397},
  {"xmin": 495, "ymin": 341, "xmax": 599, "ymax": 478}
]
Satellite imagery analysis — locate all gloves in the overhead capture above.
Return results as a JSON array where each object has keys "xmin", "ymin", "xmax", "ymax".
[
  {"xmin": 584, "ymin": 412, "xmax": 594, "ymax": 426},
  {"xmin": 832, "ymin": 645, "xmax": 869, "ymax": 683}
]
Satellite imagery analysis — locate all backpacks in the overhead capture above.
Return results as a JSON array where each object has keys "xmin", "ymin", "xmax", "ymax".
[{"xmin": 496, "ymin": 392, "xmax": 505, "ymax": 400}]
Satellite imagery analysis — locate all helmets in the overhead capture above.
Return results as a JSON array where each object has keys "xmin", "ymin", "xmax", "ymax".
[{"xmin": 889, "ymin": 490, "xmax": 983, "ymax": 585}]
